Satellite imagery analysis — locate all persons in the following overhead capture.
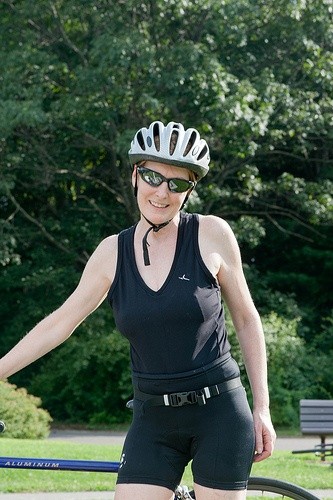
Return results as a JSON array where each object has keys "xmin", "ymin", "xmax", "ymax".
[{"xmin": 0, "ymin": 120, "xmax": 277, "ymax": 500}]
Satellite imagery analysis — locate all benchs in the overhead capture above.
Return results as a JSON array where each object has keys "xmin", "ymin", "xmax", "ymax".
[{"xmin": 299, "ymin": 399, "xmax": 333, "ymax": 462}]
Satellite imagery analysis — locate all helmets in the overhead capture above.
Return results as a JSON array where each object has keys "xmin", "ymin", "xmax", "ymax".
[{"xmin": 129, "ymin": 121, "xmax": 210, "ymax": 181}]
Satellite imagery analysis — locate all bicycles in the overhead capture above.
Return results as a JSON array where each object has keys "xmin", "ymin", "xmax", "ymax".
[{"xmin": 0, "ymin": 421, "xmax": 319, "ymax": 500}]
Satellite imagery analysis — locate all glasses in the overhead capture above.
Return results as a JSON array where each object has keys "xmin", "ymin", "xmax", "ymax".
[{"xmin": 136, "ymin": 165, "xmax": 194, "ymax": 193}]
